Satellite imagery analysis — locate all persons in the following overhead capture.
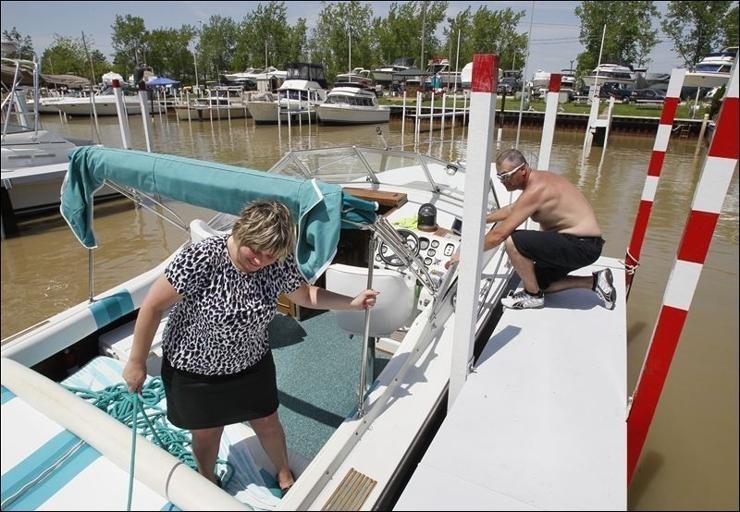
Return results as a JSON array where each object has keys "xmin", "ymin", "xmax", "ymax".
[
  {"xmin": 121, "ymin": 196, "xmax": 381, "ymax": 497},
  {"xmin": 444, "ymin": 148, "xmax": 617, "ymax": 311}
]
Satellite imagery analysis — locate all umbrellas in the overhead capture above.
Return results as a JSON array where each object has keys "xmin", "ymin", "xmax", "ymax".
[{"xmin": 145, "ymin": 76, "xmax": 181, "ymax": 89}]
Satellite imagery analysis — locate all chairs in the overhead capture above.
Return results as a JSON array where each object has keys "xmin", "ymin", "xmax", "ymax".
[
  {"xmin": 326, "ymin": 262, "xmax": 416, "ymax": 397},
  {"xmin": 190, "ymin": 218, "xmax": 234, "ymax": 245}
]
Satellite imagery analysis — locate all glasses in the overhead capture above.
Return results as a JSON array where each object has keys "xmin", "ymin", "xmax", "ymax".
[{"xmin": 496, "ymin": 161, "xmax": 527, "ymax": 182}]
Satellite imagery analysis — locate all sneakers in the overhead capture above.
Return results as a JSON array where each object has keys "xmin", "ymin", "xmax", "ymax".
[
  {"xmin": 590, "ymin": 268, "xmax": 617, "ymax": 311},
  {"xmin": 500, "ymin": 288, "xmax": 545, "ymax": 310}
]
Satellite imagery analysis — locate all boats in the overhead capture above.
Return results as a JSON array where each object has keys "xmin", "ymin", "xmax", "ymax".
[{"xmin": 1, "ymin": 143, "xmax": 538, "ymax": 511}]
